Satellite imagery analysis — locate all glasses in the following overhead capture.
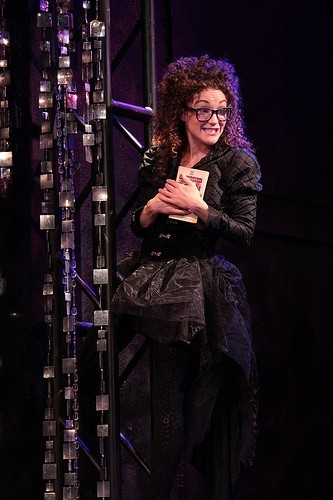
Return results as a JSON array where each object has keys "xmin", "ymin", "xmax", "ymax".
[{"xmin": 182, "ymin": 104, "xmax": 233, "ymax": 122}]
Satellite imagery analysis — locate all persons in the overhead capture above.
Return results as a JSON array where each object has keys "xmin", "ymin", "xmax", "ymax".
[{"xmin": 102, "ymin": 49, "xmax": 263, "ymax": 499}]
